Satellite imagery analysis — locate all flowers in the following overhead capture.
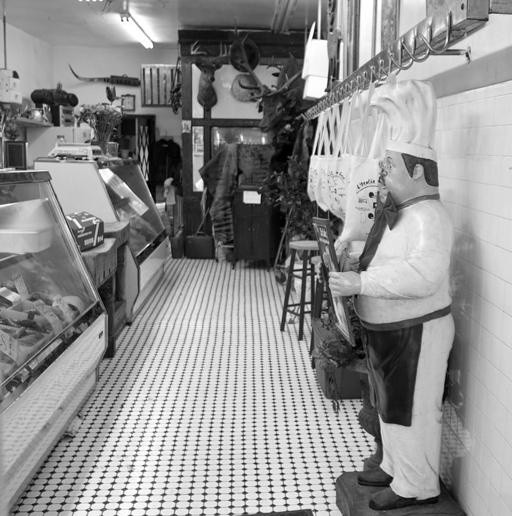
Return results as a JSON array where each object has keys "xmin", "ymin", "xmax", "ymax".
[{"xmin": 75, "ymin": 103, "xmax": 124, "ymax": 137}]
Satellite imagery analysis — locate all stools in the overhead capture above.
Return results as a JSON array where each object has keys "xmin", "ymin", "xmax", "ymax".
[{"xmin": 280, "ymin": 240, "xmax": 320, "ymax": 341}]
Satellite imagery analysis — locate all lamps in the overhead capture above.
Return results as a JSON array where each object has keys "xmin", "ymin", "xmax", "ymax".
[{"xmin": 120, "ymin": 11, "xmax": 154, "ymax": 50}]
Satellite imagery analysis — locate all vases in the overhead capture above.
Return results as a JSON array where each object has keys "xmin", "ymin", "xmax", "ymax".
[{"xmin": 96, "ymin": 139, "xmax": 108, "ymax": 154}]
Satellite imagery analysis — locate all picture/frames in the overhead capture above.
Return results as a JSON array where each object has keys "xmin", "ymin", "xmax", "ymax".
[{"xmin": 121, "ymin": 94, "xmax": 135, "ymax": 111}]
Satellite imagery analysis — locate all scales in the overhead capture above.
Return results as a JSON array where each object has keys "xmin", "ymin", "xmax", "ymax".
[{"xmin": 48, "ymin": 143, "xmax": 105, "ymax": 160}]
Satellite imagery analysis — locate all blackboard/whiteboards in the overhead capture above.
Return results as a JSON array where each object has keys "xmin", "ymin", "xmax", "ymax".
[{"xmin": 312, "ymin": 217, "xmax": 356, "ymax": 347}]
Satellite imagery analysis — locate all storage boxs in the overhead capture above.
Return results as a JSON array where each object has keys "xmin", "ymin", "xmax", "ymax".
[{"xmin": 66, "ymin": 211, "xmax": 104, "ymax": 252}]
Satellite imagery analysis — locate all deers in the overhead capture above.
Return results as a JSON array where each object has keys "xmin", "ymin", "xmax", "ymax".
[
  {"xmin": 190, "ymin": 39, "xmax": 232, "ymax": 107},
  {"xmin": 232, "ymin": 20, "xmax": 303, "ymax": 132}
]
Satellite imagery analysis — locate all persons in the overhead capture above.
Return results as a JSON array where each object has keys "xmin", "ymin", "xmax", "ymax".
[{"xmin": 325, "ymin": 78, "xmax": 460, "ymax": 512}]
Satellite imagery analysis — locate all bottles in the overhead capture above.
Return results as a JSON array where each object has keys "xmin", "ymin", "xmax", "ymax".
[{"xmin": 56, "ymin": 136, "xmax": 67, "ymax": 160}]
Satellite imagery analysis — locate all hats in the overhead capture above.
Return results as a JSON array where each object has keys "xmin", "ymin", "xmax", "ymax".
[
  {"xmin": 231, "ymin": 74, "xmax": 260, "ymax": 102},
  {"xmin": 229, "ymin": 37, "xmax": 259, "ymax": 72}
]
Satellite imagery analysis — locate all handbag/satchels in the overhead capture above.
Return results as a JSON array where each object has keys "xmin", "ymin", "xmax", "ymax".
[
  {"xmin": 306, "ymin": 74, "xmax": 374, "ymax": 220},
  {"xmin": 339, "ymin": 112, "xmax": 388, "ymax": 244}
]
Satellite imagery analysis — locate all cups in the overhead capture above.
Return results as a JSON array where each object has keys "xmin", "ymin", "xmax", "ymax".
[{"xmin": 120, "ymin": 149, "xmax": 129, "ymax": 160}]
